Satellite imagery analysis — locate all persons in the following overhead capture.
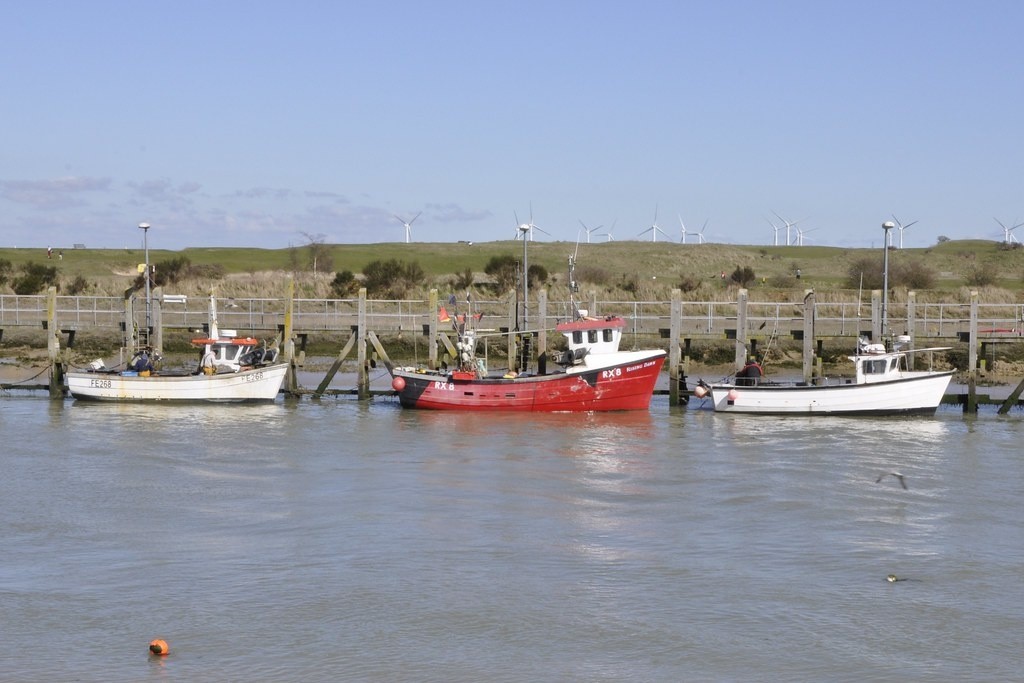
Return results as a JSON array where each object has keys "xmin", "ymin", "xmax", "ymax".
[
  {"xmin": 59, "ymin": 251, "xmax": 62, "ymax": 260},
  {"xmin": 136, "ymin": 354, "xmax": 153, "ymax": 377},
  {"xmin": 201, "ymin": 350, "xmax": 218, "ymax": 376},
  {"xmin": 796, "ymin": 268, "xmax": 800, "ymax": 279},
  {"xmin": 48, "ymin": 246, "xmax": 51, "ymax": 259}
]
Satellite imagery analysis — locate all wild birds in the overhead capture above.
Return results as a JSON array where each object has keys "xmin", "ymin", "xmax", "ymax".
[
  {"xmin": 874, "ymin": 469, "xmax": 909, "ymax": 490},
  {"xmin": 883, "ymin": 573, "xmax": 922, "ymax": 584}
]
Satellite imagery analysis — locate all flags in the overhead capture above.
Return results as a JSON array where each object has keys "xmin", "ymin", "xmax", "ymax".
[{"xmin": 439, "ymin": 294, "xmax": 482, "ymax": 325}]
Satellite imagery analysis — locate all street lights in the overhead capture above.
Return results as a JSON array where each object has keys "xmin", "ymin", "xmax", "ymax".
[
  {"xmin": 138, "ymin": 223, "xmax": 150, "ymax": 331},
  {"xmin": 882, "ymin": 221, "xmax": 895, "ymax": 334},
  {"xmin": 520, "ymin": 224, "xmax": 529, "ymax": 331}
]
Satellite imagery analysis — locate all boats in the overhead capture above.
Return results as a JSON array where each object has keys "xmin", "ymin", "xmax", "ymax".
[
  {"xmin": 67, "ymin": 337, "xmax": 289, "ymax": 401},
  {"xmin": 392, "ymin": 314, "xmax": 668, "ymax": 413},
  {"xmin": 694, "ymin": 352, "xmax": 958, "ymax": 420}
]
